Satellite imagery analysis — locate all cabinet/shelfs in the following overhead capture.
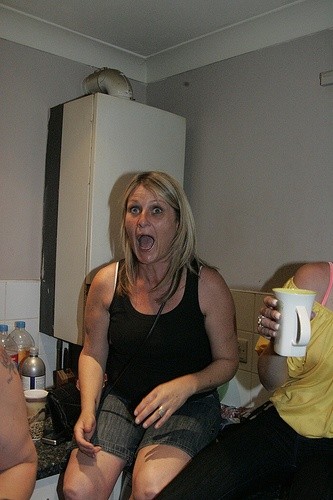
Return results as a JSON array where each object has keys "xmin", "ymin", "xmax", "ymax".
[{"xmin": 53, "ymin": 94, "xmax": 187, "ymax": 345}]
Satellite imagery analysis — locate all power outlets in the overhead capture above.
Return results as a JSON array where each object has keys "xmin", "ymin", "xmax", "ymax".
[{"xmin": 237, "ymin": 337, "xmax": 248, "ymax": 364}]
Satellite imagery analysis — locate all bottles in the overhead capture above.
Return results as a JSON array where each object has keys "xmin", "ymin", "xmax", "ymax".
[
  {"xmin": 21, "ymin": 346, "xmax": 47, "ymax": 391},
  {"xmin": 7, "ymin": 321, "xmax": 36, "ymax": 369},
  {"xmin": 0, "ymin": 325, "xmax": 19, "ymax": 368}
]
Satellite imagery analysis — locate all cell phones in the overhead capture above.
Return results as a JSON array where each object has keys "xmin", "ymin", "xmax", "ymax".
[{"xmin": 42, "ymin": 431, "xmax": 66, "ymax": 446}]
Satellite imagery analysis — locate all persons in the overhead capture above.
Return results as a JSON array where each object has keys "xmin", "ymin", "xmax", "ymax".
[
  {"xmin": 0, "ymin": 342, "xmax": 39, "ymax": 500},
  {"xmin": 151, "ymin": 261, "xmax": 333, "ymax": 500},
  {"xmin": 63, "ymin": 172, "xmax": 240, "ymax": 500}
]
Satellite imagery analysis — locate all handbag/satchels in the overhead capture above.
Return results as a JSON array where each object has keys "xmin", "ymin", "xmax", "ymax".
[{"xmin": 42, "ymin": 383, "xmax": 83, "ymax": 434}]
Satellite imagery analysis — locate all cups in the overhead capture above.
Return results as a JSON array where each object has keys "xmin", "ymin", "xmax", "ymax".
[
  {"xmin": 272, "ymin": 286, "xmax": 318, "ymax": 359},
  {"xmin": 24, "ymin": 389, "xmax": 49, "ymax": 442}
]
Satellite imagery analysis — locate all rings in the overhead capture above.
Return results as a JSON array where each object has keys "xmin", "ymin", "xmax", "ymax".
[
  {"xmin": 257, "ymin": 315, "xmax": 265, "ymax": 327},
  {"xmin": 158, "ymin": 406, "xmax": 165, "ymax": 417}
]
